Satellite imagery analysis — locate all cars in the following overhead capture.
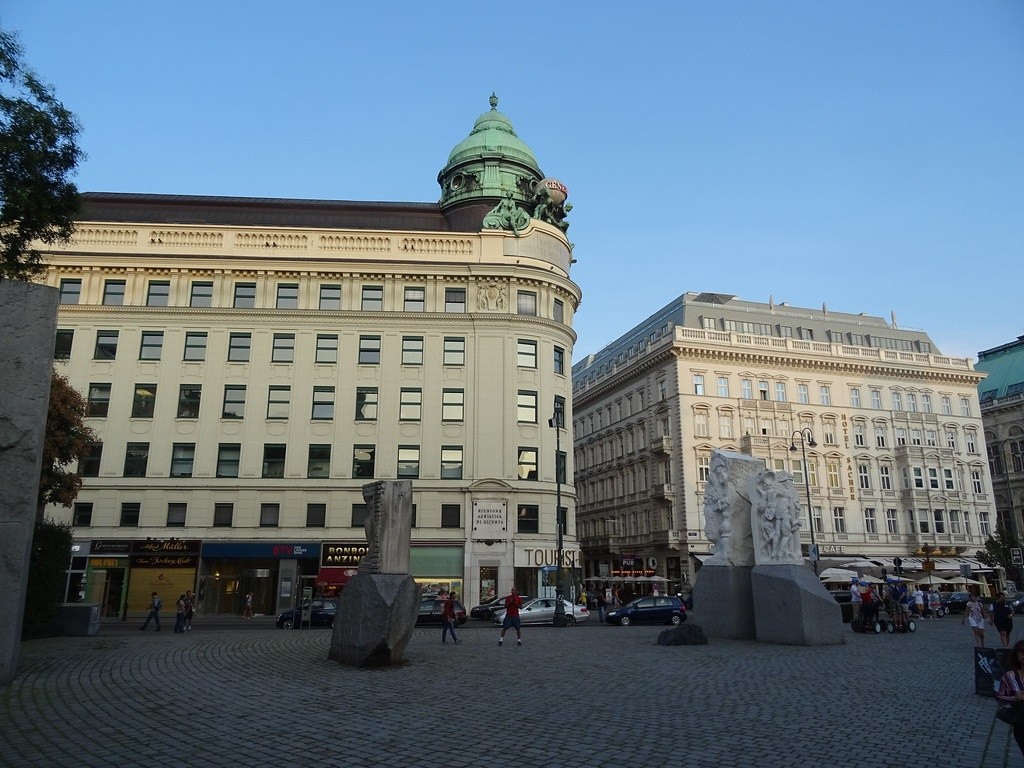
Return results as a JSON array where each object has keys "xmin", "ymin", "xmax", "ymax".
[
  {"xmin": 276, "ymin": 599, "xmax": 336, "ymax": 630},
  {"xmin": 419, "ymin": 600, "xmax": 467, "ymax": 628},
  {"xmin": 901, "ymin": 592, "xmax": 1024, "ymax": 618},
  {"xmin": 604, "ymin": 596, "xmax": 687, "ymax": 626},
  {"xmin": 491, "ymin": 598, "xmax": 589, "ymax": 627},
  {"xmin": 470, "ymin": 595, "xmax": 529, "ymax": 621}
]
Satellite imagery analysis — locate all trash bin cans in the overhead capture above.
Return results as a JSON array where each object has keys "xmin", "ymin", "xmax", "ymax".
[{"xmin": 57, "ymin": 602, "xmax": 102, "ymax": 637}]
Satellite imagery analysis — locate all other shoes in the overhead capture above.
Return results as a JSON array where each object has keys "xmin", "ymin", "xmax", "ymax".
[
  {"xmin": 139, "ymin": 627, "xmax": 144, "ymax": 631},
  {"xmin": 499, "ymin": 641, "xmax": 502, "ymax": 646},
  {"xmin": 455, "ymin": 639, "xmax": 462, "ymax": 645},
  {"xmin": 518, "ymin": 642, "xmax": 521, "ymax": 646},
  {"xmin": 442, "ymin": 642, "xmax": 448, "ymax": 645},
  {"xmin": 155, "ymin": 628, "xmax": 161, "ymax": 631}
]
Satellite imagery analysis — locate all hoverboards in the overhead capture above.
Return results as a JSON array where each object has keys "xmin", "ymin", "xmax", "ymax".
[{"xmin": 851, "ymin": 599, "xmax": 917, "ymax": 633}]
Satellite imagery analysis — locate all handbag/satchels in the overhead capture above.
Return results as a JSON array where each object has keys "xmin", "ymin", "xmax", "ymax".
[
  {"xmin": 977, "ymin": 601, "xmax": 986, "ymax": 618},
  {"xmin": 997, "ymin": 670, "xmax": 1024, "ymax": 728}
]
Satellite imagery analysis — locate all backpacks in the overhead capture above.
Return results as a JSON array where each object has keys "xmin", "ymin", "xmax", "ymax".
[{"xmin": 890, "ymin": 586, "xmax": 899, "ymax": 600}]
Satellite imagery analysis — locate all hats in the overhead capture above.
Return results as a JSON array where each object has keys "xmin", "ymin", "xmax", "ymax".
[{"xmin": 852, "ymin": 578, "xmax": 859, "ymax": 583}]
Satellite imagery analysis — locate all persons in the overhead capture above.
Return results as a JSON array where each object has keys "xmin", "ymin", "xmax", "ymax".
[
  {"xmin": 138, "ymin": 592, "xmax": 161, "ymax": 631},
  {"xmin": 489, "ymin": 189, "xmax": 520, "ymax": 236},
  {"xmin": 242, "ymin": 592, "xmax": 254, "ymax": 620},
  {"xmin": 498, "ymin": 588, "xmax": 522, "ymax": 646},
  {"xmin": 440, "ymin": 591, "xmax": 462, "ymax": 645},
  {"xmin": 539, "ymin": 199, "xmax": 572, "ymax": 232},
  {"xmin": 173, "ymin": 591, "xmax": 195, "ymax": 633},
  {"xmin": 961, "ymin": 593, "xmax": 988, "ymax": 648},
  {"xmin": 850, "ymin": 578, "xmax": 939, "ymax": 623},
  {"xmin": 990, "ymin": 592, "xmax": 1013, "ymax": 648},
  {"xmin": 1002, "ymin": 587, "xmax": 1009, "ymax": 600},
  {"xmin": 577, "ymin": 588, "xmax": 623, "ymax": 610}
]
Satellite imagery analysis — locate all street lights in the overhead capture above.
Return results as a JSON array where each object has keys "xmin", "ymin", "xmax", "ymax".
[{"xmin": 790, "ymin": 428, "xmax": 818, "ymax": 577}]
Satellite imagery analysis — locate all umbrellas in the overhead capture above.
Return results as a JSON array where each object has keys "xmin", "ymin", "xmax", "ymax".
[
  {"xmin": 583, "ymin": 576, "xmax": 672, "ymax": 595},
  {"xmin": 820, "ymin": 571, "xmax": 984, "ymax": 593}
]
